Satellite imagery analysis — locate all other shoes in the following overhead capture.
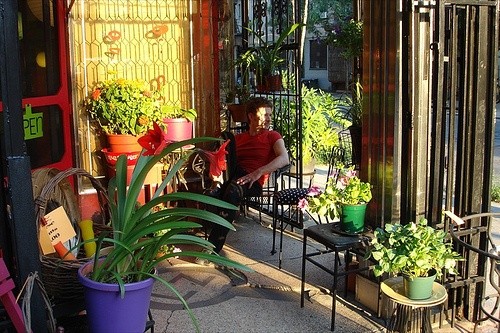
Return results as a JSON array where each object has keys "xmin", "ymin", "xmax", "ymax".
[{"xmin": 195, "ymin": 248, "xmax": 212, "ymax": 266}]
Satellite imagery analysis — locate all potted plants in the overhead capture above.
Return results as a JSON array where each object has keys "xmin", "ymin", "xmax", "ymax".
[
  {"xmin": 160, "ymin": 103, "xmax": 197, "ymax": 142},
  {"xmin": 228, "ymin": 24, "xmax": 306, "ymax": 123},
  {"xmin": 361, "ymin": 218, "xmax": 459, "ymax": 299},
  {"xmin": 326, "ymin": 82, "xmax": 362, "ymax": 166}
]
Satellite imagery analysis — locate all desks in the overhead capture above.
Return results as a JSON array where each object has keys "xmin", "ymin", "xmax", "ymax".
[
  {"xmin": 301, "ymin": 221, "xmax": 381, "ymax": 330},
  {"xmin": 380, "ymin": 276, "xmax": 448, "ymax": 333}
]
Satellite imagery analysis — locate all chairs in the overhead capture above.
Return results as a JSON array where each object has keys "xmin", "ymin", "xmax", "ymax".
[
  {"xmin": 205, "ymin": 165, "xmax": 292, "ymax": 253},
  {"xmin": 218, "ymin": 125, "xmax": 345, "ymax": 269}
]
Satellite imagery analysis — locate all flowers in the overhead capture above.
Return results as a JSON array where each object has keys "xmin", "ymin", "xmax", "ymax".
[
  {"xmin": 54, "ymin": 137, "xmax": 252, "ymax": 333},
  {"xmin": 298, "ymin": 167, "xmax": 372, "ymax": 219},
  {"xmin": 83, "ymin": 80, "xmax": 156, "ymax": 135}
]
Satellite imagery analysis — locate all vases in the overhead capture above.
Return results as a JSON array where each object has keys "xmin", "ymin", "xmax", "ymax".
[
  {"xmin": 78, "ymin": 257, "xmax": 157, "ymax": 333},
  {"xmin": 107, "ymin": 133, "xmax": 143, "ymax": 152},
  {"xmin": 339, "ymin": 204, "xmax": 367, "ymax": 233}
]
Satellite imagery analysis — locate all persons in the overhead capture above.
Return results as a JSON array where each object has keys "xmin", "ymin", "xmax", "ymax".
[{"xmin": 194, "ymin": 98, "xmax": 289, "ymax": 265}]
